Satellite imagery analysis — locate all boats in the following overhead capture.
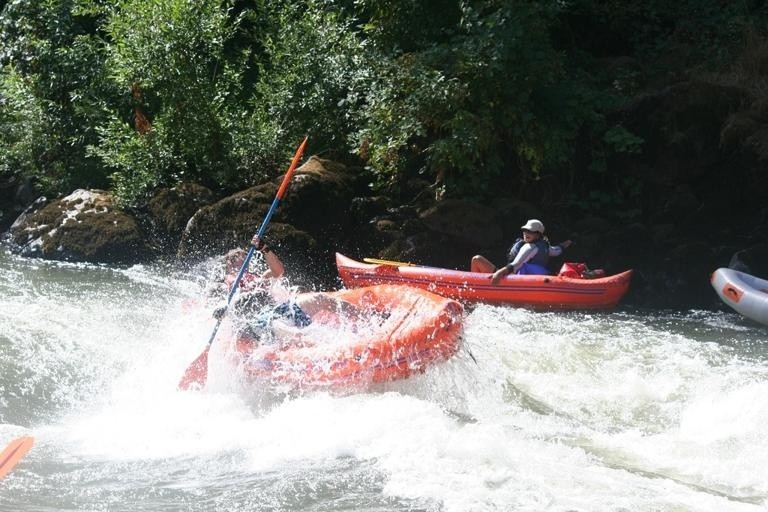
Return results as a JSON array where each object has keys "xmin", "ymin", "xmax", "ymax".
[
  {"xmin": 710, "ymin": 267, "xmax": 768, "ymax": 327},
  {"xmin": 219, "ymin": 284, "xmax": 466, "ymax": 412},
  {"xmin": 334, "ymin": 250, "xmax": 636, "ymax": 314}
]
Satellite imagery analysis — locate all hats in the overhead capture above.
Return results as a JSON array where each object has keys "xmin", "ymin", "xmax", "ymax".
[{"xmin": 521, "ymin": 219, "xmax": 545, "ymax": 234}]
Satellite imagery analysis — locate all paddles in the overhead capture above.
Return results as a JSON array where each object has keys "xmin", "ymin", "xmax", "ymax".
[
  {"xmin": 0, "ymin": 435, "xmax": 33, "ymax": 486},
  {"xmin": 364, "ymin": 257, "xmax": 431, "ymax": 267},
  {"xmin": 177, "ymin": 138, "xmax": 307, "ymax": 392}
]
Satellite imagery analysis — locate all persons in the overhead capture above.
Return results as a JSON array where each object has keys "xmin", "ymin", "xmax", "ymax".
[
  {"xmin": 471, "ymin": 219, "xmax": 573, "ymax": 287},
  {"xmin": 212, "ymin": 235, "xmax": 390, "ymax": 354}
]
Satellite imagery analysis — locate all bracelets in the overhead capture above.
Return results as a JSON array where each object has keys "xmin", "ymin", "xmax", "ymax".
[
  {"xmin": 503, "ymin": 264, "xmax": 513, "ymax": 273},
  {"xmin": 259, "ymin": 245, "xmax": 270, "ymax": 254}
]
